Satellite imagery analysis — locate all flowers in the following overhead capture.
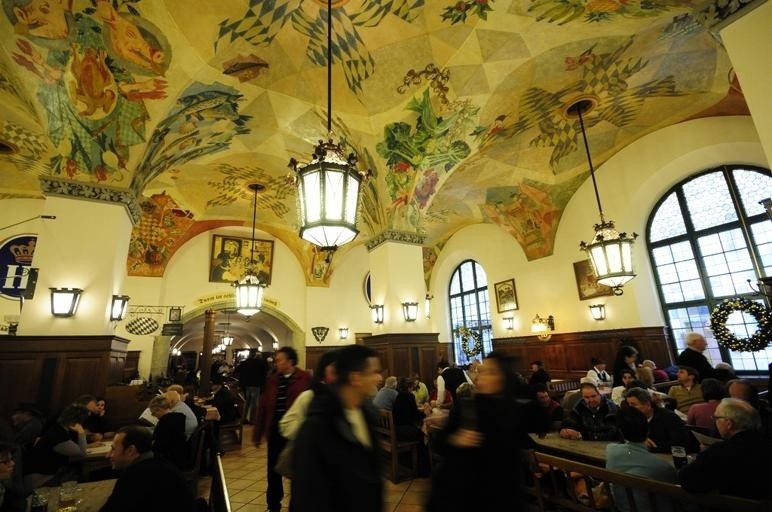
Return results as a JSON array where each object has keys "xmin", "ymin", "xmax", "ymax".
[
  {"xmin": 461, "ymin": 328, "xmax": 483, "ymax": 359},
  {"xmin": 709, "ymin": 296, "xmax": 772, "ymax": 353}
]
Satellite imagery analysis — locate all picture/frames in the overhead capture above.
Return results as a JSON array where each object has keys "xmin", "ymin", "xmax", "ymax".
[
  {"xmin": 209, "ymin": 234, "xmax": 274, "ymax": 286},
  {"xmin": 494, "ymin": 278, "xmax": 519, "ymax": 313},
  {"xmin": 573, "ymin": 259, "xmax": 614, "ymax": 302}
]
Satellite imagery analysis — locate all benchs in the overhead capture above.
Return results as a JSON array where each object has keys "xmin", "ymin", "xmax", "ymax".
[
  {"xmin": 650, "ymin": 379, "xmax": 681, "ymax": 394},
  {"xmin": 546, "ymin": 376, "xmax": 586, "ymax": 404}
]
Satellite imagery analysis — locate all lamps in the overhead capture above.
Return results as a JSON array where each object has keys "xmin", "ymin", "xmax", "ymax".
[
  {"xmin": 589, "ymin": 304, "xmax": 605, "ymax": 320},
  {"xmin": 221, "ymin": 323, "xmax": 234, "ymax": 346},
  {"xmin": 561, "ymin": 93, "xmax": 638, "ymax": 296},
  {"xmin": 49, "ymin": 286, "xmax": 83, "ymax": 318},
  {"xmin": 287, "ymin": 0, "xmax": 372, "ymax": 261},
  {"xmin": 228, "ymin": 180, "xmax": 271, "ymax": 319},
  {"xmin": 110, "ymin": 294, "xmax": 131, "ymax": 321},
  {"xmin": 401, "ymin": 301, "xmax": 418, "ymax": 321},
  {"xmin": 338, "ymin": 328, "xmax": 348, "ymax": 340},
  {"xmin": 503, "ymin": 317, "xmax": 514, "ymax": 329},
  {"xmin": 369, "ymin": 305, "xmax": 384, "ymax": 324},
  {"xmin": 530, "ymin": 313, "xmax": 555, "ymax": 333}
]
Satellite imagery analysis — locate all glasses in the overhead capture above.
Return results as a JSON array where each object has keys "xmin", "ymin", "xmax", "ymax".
[{"xmin": 711, "ymin": 415, "xmax": 727, "ymax": 423}]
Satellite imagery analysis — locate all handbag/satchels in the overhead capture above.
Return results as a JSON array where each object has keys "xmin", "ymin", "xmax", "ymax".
[{"xmin": 272, "ymin": 440, "xmax": 296, "ymax": 479}]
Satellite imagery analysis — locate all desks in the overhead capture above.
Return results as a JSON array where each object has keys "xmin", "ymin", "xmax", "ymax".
[
  {"xmin": 524, "ymin": 428, "xmax": 772, "ymax": 512},
  {"xmin": 194, "ymin": 396, "xmax": 221, "ymax": 420},
  {"xmin": 26, "ymin": 435, "xmax": 118, "ymax": 511},
  {"xmin": 416, "ymin": 409, "xmax": 451, "ymax": 474}
]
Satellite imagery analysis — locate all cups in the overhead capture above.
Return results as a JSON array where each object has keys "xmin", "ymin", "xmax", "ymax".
[
  {"xmin": 59, "ymin": 482, "xmax": 85, "ymax": 512},
  {"xmin": 130, "ymin": 380, "xmax": 144, "ymax": 385},
  {"xmin": 31, "ymin": 487, "xmax": 51, "ymax": 512},
  {"xmin": 671, "ymin": 446, "xmax": 694, "ymax": 470}
]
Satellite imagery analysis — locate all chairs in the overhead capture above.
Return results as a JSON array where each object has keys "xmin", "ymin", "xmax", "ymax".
[
  {"xmin": 208, "ymin": 453, "xmax": 231, "ymax": 512},
  {"xmin": 186, "ymin": 422, "xmax": 208, "ymax": 498},
  {"xmin": 217, "ymin": 376, "xmax": 246, "ymax": 449},
  {"xmin": 758, "ymin": 390, "xmax": 771, "ymax": 400},
  {"xmin": 375, "ymin": 409, "xmax": 420, "ymax": 484}
]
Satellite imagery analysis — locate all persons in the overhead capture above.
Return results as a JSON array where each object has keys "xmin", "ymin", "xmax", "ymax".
[
  {"xmin": 419, "ymin": 353, "xmax": 533, "ymax": 511},
  {"xmin": 287, "ymin": 344, "xmax": 387, "ymax": 510},
  {"xmin": 251, "ymin": 347, "xmax": 314, "ymax": 511},
  {"xmin": 371, "ymin": 359, "xmax": 478, "ymax": 482},
  {"xmin": 278, "ymin": 348, "xmax": 342, "ymax": 441}
]
